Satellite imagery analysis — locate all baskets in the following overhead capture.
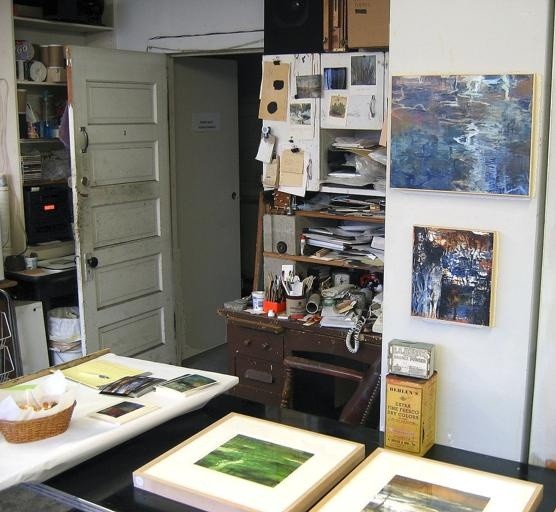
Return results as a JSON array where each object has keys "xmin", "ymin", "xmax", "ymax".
[{"xmin": 0, "ymin": 400, "xmax": 76, "ymax": 446}]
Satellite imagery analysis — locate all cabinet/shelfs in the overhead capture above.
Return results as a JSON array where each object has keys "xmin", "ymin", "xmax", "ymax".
[
  {"xmin": 252, "ymin": 189, "xmax": 384, "ymax": 292},
  {"xmin": 260, "ymin": 52, "xmax": 387, "ymax": 188},
  {"xmin": 1, "ymin": 0, "xmax": 121, "ymax": 264}
]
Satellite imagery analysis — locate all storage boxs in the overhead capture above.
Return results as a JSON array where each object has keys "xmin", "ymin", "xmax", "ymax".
[{"xmin": 346, "ymin": 1, "xmax": 389, "ymax": 50}]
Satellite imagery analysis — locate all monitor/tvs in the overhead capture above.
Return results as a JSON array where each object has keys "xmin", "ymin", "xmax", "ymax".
[{"xmin": 23, "ymin": 183, "xmax": 73, "ymax": 244}]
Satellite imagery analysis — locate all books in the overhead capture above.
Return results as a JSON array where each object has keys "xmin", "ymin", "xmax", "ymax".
[
  {"xmin": 305, "ymin": 222, "xmax": 385, "ymax": 264},
  {"xmin": 331, "ymin": 136, "xmax": 382, "ymax": 149},
  {"xmin": 49, "ymin": 360, "xmax": 140, "ymax": 391}
]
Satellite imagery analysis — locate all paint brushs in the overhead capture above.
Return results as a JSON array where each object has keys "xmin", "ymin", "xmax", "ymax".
[{"xmin": 264, "ymin": 270, "xmax": 306, "ymax": 303}]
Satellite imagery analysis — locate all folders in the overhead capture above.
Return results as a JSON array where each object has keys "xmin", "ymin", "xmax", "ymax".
[{"xmin": 302, "ymin": 226, "xmax": 384, "ymax": 263}]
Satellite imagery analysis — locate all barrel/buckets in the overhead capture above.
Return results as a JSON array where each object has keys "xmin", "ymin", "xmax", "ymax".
[{"xmin": 46, "ymin": 306, "xmax": 83, "ymax": 368}]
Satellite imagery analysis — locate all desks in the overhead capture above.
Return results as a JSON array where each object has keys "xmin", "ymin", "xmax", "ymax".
[
  {"xmin": 3, "ymin": 253, "xmax": 77, "ymax": 301},
  {"xmin": 215, "ymin": 296, "xmax": 382, "ymax": 432},
  {"xmin": 15, "ymin": 394, "xmax": 556, "ymax": 512}
]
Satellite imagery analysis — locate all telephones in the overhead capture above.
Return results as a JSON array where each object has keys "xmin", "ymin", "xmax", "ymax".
[
  {"xmin": 372, "ymin": 292, "xmax": 383, "ymax": 305},
  {"xmin": 320, "ymin": 291, "xmax": 369, "ymax": 354}
]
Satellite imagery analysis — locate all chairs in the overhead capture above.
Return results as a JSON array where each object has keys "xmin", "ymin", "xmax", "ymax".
[{"xmin": 278, "ymin": 355, "xmax": 381, "ymax": 424}]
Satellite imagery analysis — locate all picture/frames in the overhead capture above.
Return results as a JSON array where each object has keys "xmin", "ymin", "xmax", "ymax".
[
  {"xmin": 410, "ymin": 225, "xmax": 496, "ymax": 327},
  {"xmin": 309, "ymin": 447, "xmax": 543, "ymax": 512},
  {"xmin": 131, "ymin": 411, "xmax": 364, "ymax": 512},
  {"xmin": 390, "ymin": 74, "xmax": 536, "ymax": 198}
]
